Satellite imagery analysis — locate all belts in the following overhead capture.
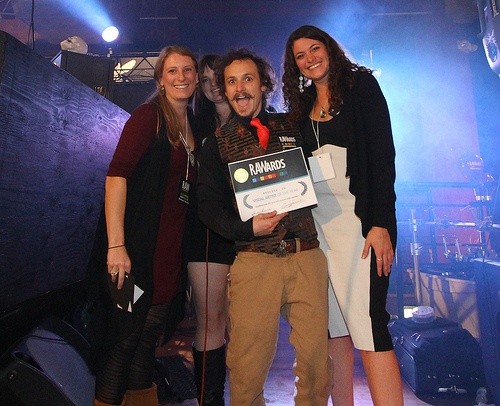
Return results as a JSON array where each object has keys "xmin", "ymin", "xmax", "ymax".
[{"xmin": 242, "ymin": 241, "xmax": 315, "ymax": 254}]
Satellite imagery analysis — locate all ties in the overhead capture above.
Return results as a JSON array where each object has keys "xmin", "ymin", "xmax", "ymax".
[{"xmin": 251, "ymin": 117, "xmax": 271, "ymax": 151}]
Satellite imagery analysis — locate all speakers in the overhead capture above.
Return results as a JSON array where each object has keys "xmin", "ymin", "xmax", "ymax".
[{"xmin": 0, "ymin": 317, "xmax": 97, "ymax": 406}]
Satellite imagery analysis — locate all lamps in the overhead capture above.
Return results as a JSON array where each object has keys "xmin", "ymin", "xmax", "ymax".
[{"xmin": 102, "ymin": 25, "xmax": 123, "ymax": 50}]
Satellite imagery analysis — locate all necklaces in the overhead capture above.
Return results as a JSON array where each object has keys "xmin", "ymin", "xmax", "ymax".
[
  {"xmin": 319, "ymin": 106, "xmax": 327, "ymax": 118},
  {"xmin": 178, "ymin": 130, "xmax": 195, "ymax": 166}
]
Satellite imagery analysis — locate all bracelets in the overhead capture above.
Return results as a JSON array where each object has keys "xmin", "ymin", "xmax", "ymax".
[{"xmin": 108, "ymin": 245, "xmax": 125, "ymax": 249}]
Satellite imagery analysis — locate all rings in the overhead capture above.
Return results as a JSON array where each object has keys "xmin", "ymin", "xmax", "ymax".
[
  {"xmin": 110, "ymin": 271, "xmax": 119, "ymax": 277},
  {"xmin": 376, "ymin": 258, "xmax": 382, "ymax": 261}
]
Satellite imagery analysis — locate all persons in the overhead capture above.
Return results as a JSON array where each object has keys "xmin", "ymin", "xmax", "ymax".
[{"xmin": 87, "ymin": 24, "xmax": 403, "ymax": 406}]
